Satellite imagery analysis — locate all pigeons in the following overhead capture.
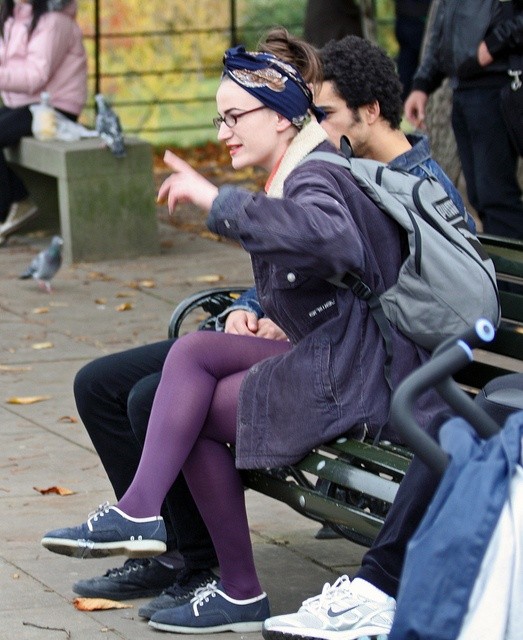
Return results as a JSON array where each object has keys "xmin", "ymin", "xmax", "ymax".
[
  {"xmin": 15, "ymin": 235, "xmax": 63, "ymax": 293},
  {"xmin": 93, "ymin": 93, "xmax": 126, "ymax": 161}
]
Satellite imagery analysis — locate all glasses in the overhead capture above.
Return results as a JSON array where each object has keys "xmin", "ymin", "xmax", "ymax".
[{"xmin": 212, "ymin": 105, "xmax": 266, "ymax": 128}]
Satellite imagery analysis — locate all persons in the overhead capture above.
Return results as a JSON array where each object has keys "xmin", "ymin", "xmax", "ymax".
[
  {"xmin": 402, "ymin": 0, "xmax": 522, "ymax": 240},
  {"xmin": 69, "ymin": 34, "xmax": 476, "ymax": 622},
  {"xmin": 455, "ymin": 2, "xmax": 522, "ymax": 160},
  {"xmin": 261, "ymin": 406, "xmax": 458, "ymax": 640},
  {"xmin": 41, "ymin": 24, "xmax": 450, "ymax": 634},
  {"xmin": 0, "ymin": 0, "xmax": 86, "ymax": 246}
]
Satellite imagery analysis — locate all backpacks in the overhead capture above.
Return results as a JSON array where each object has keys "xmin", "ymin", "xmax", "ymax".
[{"xmin": 297, "ymin": 151, "xmax": 502, "ymax": 445}]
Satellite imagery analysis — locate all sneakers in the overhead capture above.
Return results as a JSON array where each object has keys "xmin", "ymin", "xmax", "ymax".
[
  {"xmin": 40, "ymin": 501, "xmax": 167, "ymax": 559},
  {"xmin": 0, "ymin": 196, "xmax": 38, "ymax": 233},
  {"xmin": 138, "ymin": 569, "xmax": 220, "ymax": 618},
  {"xmin": 147, "ymin": 580, "xmax": 269, "ymax": 634},
  {"xmin": 73, "ymin": 559, "xmax": 184, "ymax": 599},
  {"xmin": 262, "ymin": 575, "xmax": 396, "ymax": 640}
]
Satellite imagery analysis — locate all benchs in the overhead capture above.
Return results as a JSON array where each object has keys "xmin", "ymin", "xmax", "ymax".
[
  {"xmin": 165, "ymin": 233, "xmax": 522, "ymax": 545},
  {"xmin": 3, "ymin": 133, "xmax": 159, "ymax": 266}
]
